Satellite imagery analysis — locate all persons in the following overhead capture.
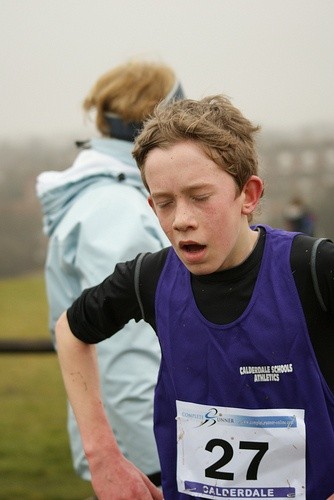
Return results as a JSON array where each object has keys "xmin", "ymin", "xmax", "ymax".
[
  {"xmin": 287, "ymin": 197, "xmax": 313, "ymax": 237},
  {"xmin": 35, "ymin": 64, "xmax": 185, "ymax": 500},
  {"xmin": 54, "ymin": 94, "xmax": 334, "ymax": 500}
]
